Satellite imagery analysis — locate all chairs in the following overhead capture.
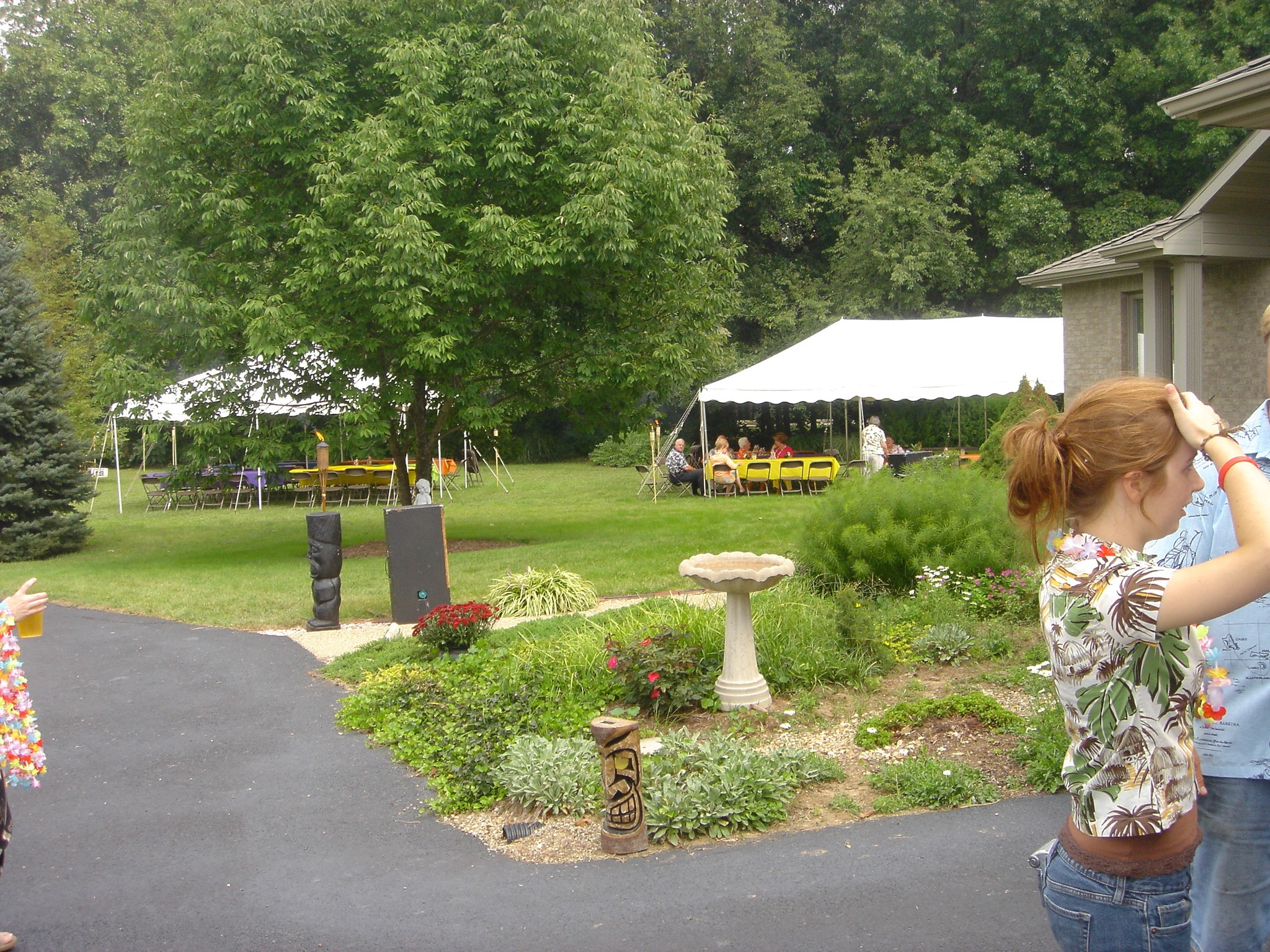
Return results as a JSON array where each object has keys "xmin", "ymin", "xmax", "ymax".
[
  {"xmin": 140, "ymin": 449, "xmax": 483, "ymax": 511},
  {"xmin": 634, "ymin": 449, "xmax": 869, "ymax": 501}
]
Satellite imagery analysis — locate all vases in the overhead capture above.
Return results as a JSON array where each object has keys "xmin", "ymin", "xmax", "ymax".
[{"xmin": 438, "ymin": 644, "xmax": 470, "ymax": 661}]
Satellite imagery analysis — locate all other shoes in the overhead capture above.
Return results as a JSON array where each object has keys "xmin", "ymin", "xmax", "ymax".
[
  {"xmin": 692, "ymin": 492, "xmax": 701, "ymax": 496},
  {"xmin": 701, "ymin": 492, "xmax": 709, "ymax": 497}
]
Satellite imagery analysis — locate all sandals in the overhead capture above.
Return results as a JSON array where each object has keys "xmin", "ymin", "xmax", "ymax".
[{"xmin": 0, "ymin": 932, "xmax": 18, "ymax": 952}]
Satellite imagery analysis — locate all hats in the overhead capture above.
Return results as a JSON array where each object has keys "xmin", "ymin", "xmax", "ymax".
[{"xmin": 1262, "ymin": 303, "xmax": 1270, "ymax": 344}]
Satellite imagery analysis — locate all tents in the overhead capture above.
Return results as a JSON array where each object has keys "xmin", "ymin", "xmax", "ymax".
[
  {"xmin": 636, "ymin": 316, "xmax": 1064, "ymax": 504},
  {"xmin": 85, "ymin": 338, "xmax": 514, "ymax": 516}
]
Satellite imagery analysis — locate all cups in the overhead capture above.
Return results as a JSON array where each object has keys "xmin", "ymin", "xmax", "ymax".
[
  {"xmin": 753, "ymin": 454, "xmax": 756, "ymax": 459},
  {"xmin": 17, "ymin": 603, "xmax": 43, "ymax": 638}
]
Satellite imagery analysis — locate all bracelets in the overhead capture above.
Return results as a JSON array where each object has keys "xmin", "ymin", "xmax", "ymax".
[
  {"xmin": 1218, "ymin": 456, "xmax": 1259, "ymax": 491},
  {"xmin": 1199, "ymin": 419, "xmax": 1246, "ymax": 461}
]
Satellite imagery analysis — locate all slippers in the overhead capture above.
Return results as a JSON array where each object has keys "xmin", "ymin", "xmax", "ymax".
[{"xmin": 740, "ymin": 489, "xmax": 750, "ymax": 495}]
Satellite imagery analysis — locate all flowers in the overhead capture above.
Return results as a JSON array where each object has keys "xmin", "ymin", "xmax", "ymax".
[{"xmin": 412, "ymin": 600, "xmax": 500, "ymax": 644}]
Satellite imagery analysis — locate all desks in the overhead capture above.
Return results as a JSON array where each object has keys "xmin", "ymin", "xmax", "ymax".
[
  {"xmin": 289, "ymin": 464, "xmax": 439, "ymax": 503},
  {"xmin": 214, "ymin": 462, "xmax": 317, "ymax": 485},
  {"xmin": 888, "ymin": 451, "xmax": 933, "ymax": 474},
  {"xmin": 140, "ymin": 471, "xmax": 284, "ymax": 507},
  {"xmin": 705, "ymin": 456, "xmax": 841, "ymax": 493},
  {"xmin": 734, "ymin": 451, "xmax": 818, "ymax": 456},
  {"xmin": 341, "ymin": 459, "xmax": 457, "ymax": 489}
]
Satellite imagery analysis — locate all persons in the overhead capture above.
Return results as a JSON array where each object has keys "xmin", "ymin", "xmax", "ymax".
[
  {"xmin": 0, "ymin": 576, "xmax": 56, "ymax": 952},
  {"xmin": 768, "ymin": 431, "xmax": 794, "ymax": 495},
  {"xmin": 996, "ymin": 371, "xmax": 1270, "ymax": 952},
  {"xmin": 883, "ymin": 434, "xmax": 907, "ymax": 467},
  {"xmin": 861, "ymin": 415, "xmax": 888, "ymax": 476},
  {"xmin": 1144, "ymin": 301, "xmax": 1270, "ymax": 952},
  {"xmin": 736, "ymin": 436, "xmax": 768, "ymax": 495},
  {"xmin": 705, "ymin": 434, "xmax": 746, "ymax": 497},
  {"xmin": 665, "ymin": 438, "xmax": 706, "ymax": 496}
]
